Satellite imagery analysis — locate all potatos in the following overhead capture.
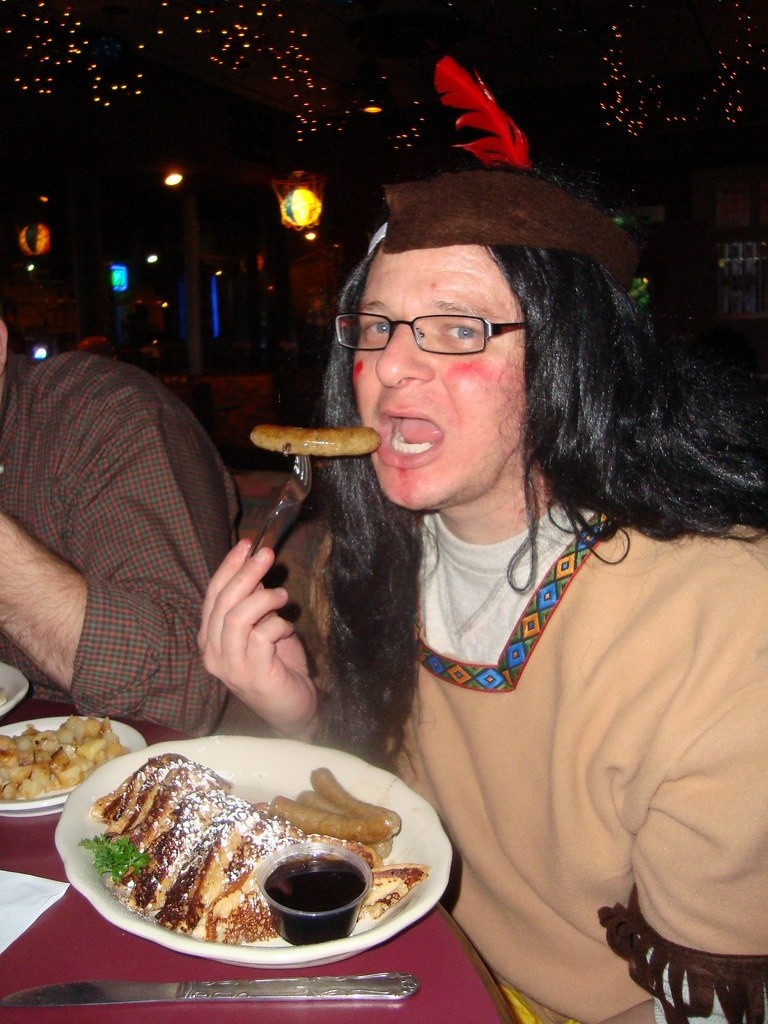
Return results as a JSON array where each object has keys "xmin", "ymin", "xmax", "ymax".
[{"xmin": 0, "ymin": 715, "xmax": 129, "ymax": 803}]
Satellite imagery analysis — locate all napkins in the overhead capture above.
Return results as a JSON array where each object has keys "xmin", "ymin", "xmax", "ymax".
[{"xmin": 0, "ymin": 869, "xmax": 70, "ymax": 951}]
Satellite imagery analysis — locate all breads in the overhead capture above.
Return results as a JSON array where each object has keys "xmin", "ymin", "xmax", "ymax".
[{"xmin": 90, "ymin": 754, "xmax": 430, "ymax": 943}]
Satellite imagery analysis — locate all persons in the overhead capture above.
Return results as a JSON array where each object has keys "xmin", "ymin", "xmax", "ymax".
[
  {"xmin": 197, "ymin": 169, "xmax": 768, "ymax": 1024},
  {"xmin": 0, "ymin": 308, "xmax": 266, "ymax": 738}
]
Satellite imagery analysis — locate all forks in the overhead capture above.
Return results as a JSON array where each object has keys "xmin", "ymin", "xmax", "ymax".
[{"xmin": 243, "ymin": 454, "xmax": 312, "ymax": 565}]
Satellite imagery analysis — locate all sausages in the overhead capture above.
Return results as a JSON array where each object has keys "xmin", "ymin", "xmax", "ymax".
[
  {"xmin": 249, "ymin": 423, "xmax": 381, "ymax": 456},
  {"xmin": 267, "ymin": 767, "xmax": 401, "ymax": 859}
]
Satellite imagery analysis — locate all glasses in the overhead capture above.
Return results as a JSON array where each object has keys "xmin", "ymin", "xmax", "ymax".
[{"xmin": 336, "ymin": 310, "xmax": 524, "ymax": 358}]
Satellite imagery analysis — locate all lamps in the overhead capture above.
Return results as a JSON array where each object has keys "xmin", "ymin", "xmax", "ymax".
[
  {"xmin": 17, "ymin": 223, "xmax": 52, "ymax": 256},
  {"xmin": 269, "ymin": 168, "xmax": 334, "ymax": 245}
]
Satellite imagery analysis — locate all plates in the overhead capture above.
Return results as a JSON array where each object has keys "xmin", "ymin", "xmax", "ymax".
[
  {"xmin": 53, "ymin": 732, "xmax": 454, "ymax": 971},
  {"xmin": 0, "ymin": 662, "xmax": 29, "ymax": 716},
  {"xmin": 0, "ymin": 715, "xmax": 148, "ymax": 817}
]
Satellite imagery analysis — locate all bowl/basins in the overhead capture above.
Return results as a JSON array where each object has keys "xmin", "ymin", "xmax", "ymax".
[{"xmin": 256, "ymin": 842, "xmax": 373, "ymax": 944}]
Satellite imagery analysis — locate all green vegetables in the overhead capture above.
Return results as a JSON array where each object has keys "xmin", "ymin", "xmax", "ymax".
[{"xmin": 79, "ymin": 834, "xmax": 150, "ymax": 884}]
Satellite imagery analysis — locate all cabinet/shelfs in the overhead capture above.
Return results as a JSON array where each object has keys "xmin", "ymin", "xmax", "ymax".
[{"xmin": 689, "ymin": 166, "xmax": 768, "ymax": 322}]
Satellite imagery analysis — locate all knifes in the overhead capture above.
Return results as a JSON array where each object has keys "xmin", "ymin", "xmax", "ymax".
[{"xmin": 1, "ymin": 971, "xmax": 419, "ymax": 1006}]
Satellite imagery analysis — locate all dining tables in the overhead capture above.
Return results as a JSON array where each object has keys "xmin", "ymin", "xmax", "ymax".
[{"xmin": 0, "ymin": 698, "xmax": 523, "ymax": 1024}]
